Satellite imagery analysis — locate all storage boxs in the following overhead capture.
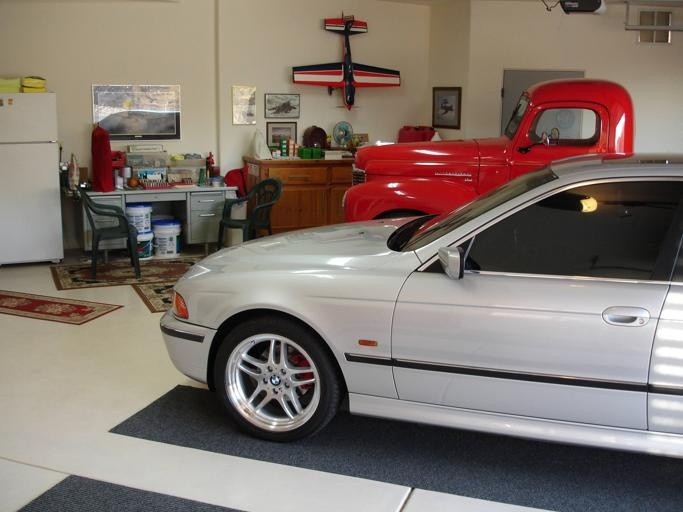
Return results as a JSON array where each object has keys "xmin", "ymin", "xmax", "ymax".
[
  {"xmin": 168, "ymin": 166, "xmax": 198, "ymax": 185},
  {"xmin": 123, "ymin": 153, "xmax": 165, "ymax": 167},
  {"xmin": 128, "ymin": 168, "xmax": 165, "ymax": 183}
]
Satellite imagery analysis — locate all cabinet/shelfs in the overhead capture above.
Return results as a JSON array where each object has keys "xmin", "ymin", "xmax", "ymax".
[
  {"xmin": 327, "ymin": 153, "xmax": 357, "ymax": 226},
  {"xmin": 241, "ymin": 155, "xmax": 329, "ymax": 235}
]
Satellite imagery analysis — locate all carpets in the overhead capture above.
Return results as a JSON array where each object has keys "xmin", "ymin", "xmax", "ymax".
[
  {"xmin": 0, "ymin": 288, "xmax": 124, "ymax": 326},
  {"xmin": 129, "ymin": 280, "xmax": 182, "ymax": 314},
  {"xmin": 16, "ymin": 475, "xmax": 240, "ymax": 510},
  {"xmin": 47, "ymin": 257, "xmax": 195, "ymax": 292},
  {"xmin": 106, "ymin": 380, "xmax": 682, "ymax": 512}
]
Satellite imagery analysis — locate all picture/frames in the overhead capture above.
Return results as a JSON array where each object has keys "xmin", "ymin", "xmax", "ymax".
[
  {"xmin": 263, "ymin": 94, "xmax": 300, "ymax": 119},
  {"xmin": 430, "ymin": 87, "xmax": 463, "ymax": 130},
  {"xmin": 265, "ymin": 121, "xmax": 298, "ymax": 150}
]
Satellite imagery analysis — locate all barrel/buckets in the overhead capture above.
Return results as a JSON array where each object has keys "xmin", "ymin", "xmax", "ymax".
[
  {"xmin": 134, "ymin": 232, "xmax": 154, "ymax": 259},
  {"xmin": 126, "ymin": 204, "xmax": 152, "ymax": 233},
  {"xmin": 152, "ymin": 220, "xmax": 182, "ymax": 256}
]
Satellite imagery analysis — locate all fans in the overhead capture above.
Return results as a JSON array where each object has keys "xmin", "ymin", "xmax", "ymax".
[{"xmin": 332, "ymin": 122, "xmax": 352, "ymax": 147}]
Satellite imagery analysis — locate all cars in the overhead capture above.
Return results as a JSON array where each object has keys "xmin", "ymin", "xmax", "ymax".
[{"xmin": 159, "ymin": 153, "xmax": 683, "ymax": 461}]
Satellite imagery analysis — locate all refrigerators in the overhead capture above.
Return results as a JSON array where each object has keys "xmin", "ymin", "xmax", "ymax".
[{"xmin": 0, "ymin": 91, "xmax": 66, "ymax": 268}]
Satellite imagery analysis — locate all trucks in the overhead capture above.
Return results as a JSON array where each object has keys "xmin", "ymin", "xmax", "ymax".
[{"xmin": 342, "ymin": 79, "xmax": 634, "ymax": 223}]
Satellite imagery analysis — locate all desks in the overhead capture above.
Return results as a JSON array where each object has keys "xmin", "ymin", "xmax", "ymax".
[{"xmin": 62, "ymin": 184, "xmax": 240, "ymax": 262}]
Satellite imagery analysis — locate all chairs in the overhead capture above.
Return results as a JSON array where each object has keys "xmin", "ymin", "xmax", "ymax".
[
  {"xmin": 217, "ymin": 177, "xmax": 280, "ymax": 251},
  {"xmin": 73, "ymin": 185, "xmax": 142, "ymax": 280}
]
